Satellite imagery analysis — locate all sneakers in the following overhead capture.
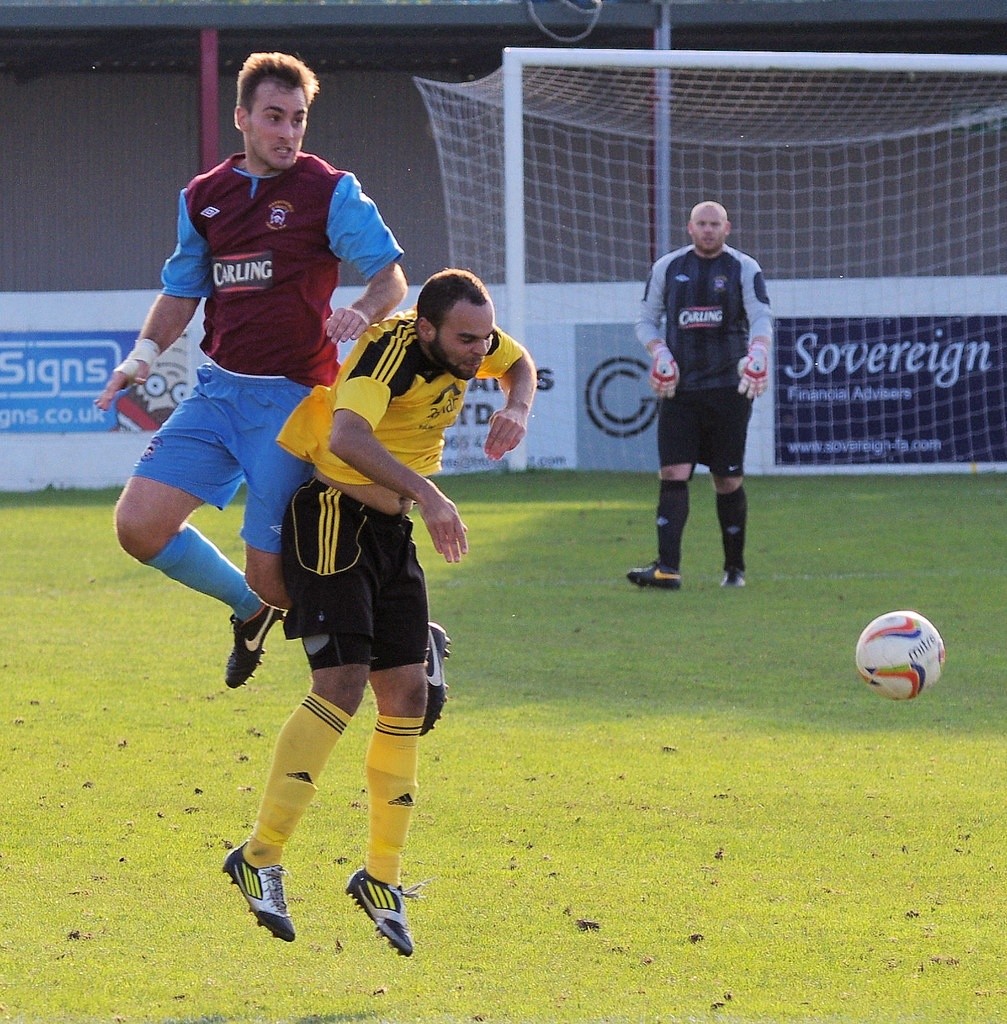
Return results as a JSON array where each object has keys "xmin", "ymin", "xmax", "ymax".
[
  {"xmin": 222, "ymin": 841, "xmax": 296, "ymax": 943},
  {"xmin": 721, "ymin": 571, "xmax": 745, "ymax": 587},
  {"xmin": 344, "ymin": 866, "xmax": 436, "ymax": 957},
  {"xmin": 225, "ymin": 603, "xmax": 287, "ymax": 689},
  {"xmin": 420, "ymin": 622, "xmax": 451, "ymax": 736},
  {"xmin": 626, "ymin": 561, "xmax": 681, "ymax": 590}
]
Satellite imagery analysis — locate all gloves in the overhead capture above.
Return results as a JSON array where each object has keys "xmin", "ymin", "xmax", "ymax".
[
  {"xmin": 649, "ymin": 342, "xmax": 680, "ymax": 399},
  {"xmin": 736, "ymin": 341, "xmax": 769, "ymax": 399}
]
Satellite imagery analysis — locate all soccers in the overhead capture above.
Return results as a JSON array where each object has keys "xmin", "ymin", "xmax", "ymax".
[{"xmin": 855, "ymin": 609, "xmax": 946, "ymax": 701}]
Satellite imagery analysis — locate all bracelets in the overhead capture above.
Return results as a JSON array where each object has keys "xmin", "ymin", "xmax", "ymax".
[{"xmin": 126, "ymin": 339, "xmax": 160, "ymax": 368}]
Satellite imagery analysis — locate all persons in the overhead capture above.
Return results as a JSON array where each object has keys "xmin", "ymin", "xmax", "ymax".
[
  {"xmin": 93, "ymin": 53, "xmax": 448, "ymax": 736},
  {"xmin": 221, "ymin": 269, "xmax": 534, "ymax": 957},
  {"xmin": 624, "ymin": 202, "xmax": 773, "ymax": 589}
]
